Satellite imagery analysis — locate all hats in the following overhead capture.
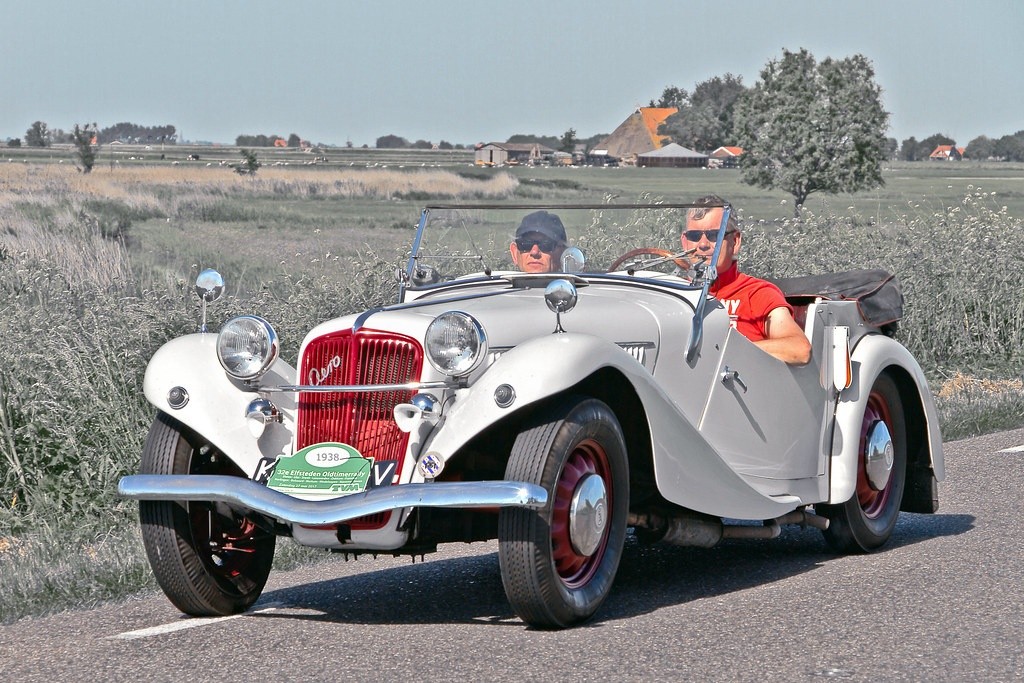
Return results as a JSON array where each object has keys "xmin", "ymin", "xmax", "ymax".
[{"xmin": 515, "ymin": 210, "xmax": 567, "ymax": 247}]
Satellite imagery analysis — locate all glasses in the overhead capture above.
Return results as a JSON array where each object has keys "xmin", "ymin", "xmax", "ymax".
[
  {"xmin": 685, "ymin": 229, "xmax": 737, "ymax": 242},
  {"xmin": 515, "ymin": 238, "xmax": 557, "ymax": 252}
]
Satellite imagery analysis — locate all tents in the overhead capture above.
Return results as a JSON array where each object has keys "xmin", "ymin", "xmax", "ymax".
[{"xmin": 637, "ymin": 142, "xmax": 708, "ymax": 168}]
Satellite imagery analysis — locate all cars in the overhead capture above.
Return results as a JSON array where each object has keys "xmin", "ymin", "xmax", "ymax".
[{"xmin": 116, "ymin": 200, "xmax": 950, "ymax": 630}]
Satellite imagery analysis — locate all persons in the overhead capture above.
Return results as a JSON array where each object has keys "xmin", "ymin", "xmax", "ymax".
[
  {"xmin": 681, "ymin": 193, "xmax": 813, "ymax": 366},
  {"xmin": 510, "ymin": 210, "xmax": 567, "ymax": 274}
]
